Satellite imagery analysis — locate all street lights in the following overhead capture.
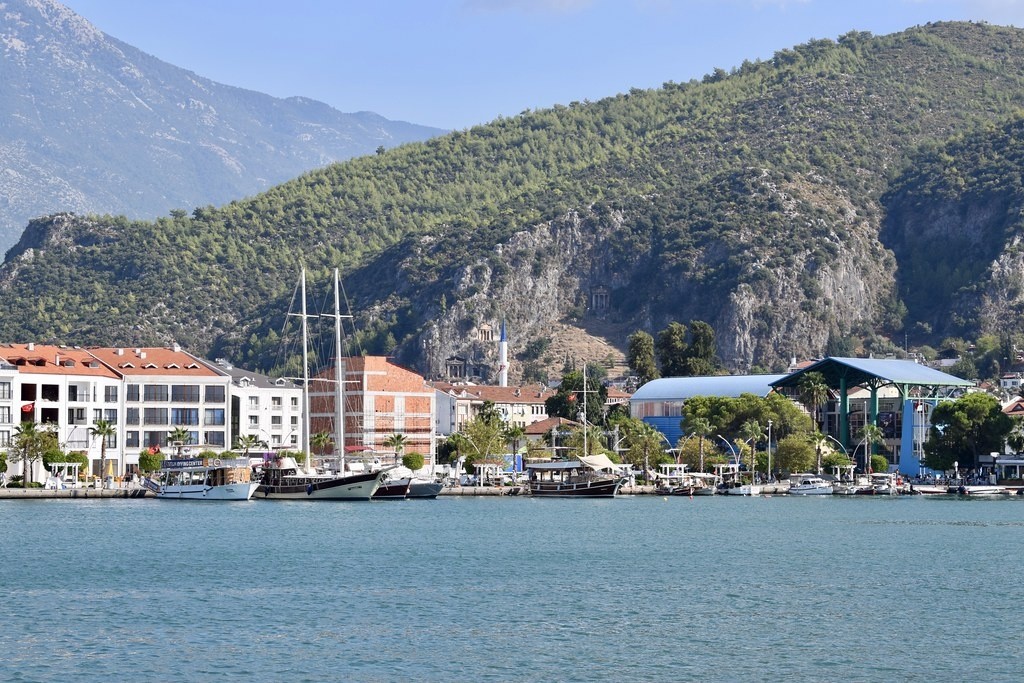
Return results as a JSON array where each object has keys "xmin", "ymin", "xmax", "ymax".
[
  {"xmin": 765, "ymin": 417, "xmax": 773, "ymax": 481},
  {"xmin": 657, "ymin": 431, "xmax": 697, "ymax": 464},
  {"xmin": 717, "ymin": 432, "xmax": 755, "ymax": 465},
  {"xmin": 826, "ymin": 434, "xmax": 871, "ymax": 461}
]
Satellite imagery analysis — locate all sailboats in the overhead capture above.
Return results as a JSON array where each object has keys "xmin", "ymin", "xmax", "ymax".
[
  {"xmin": 252, "ymin": 265, "xmax": 404, "ymax": 501},
  {"xmin": 526, "ymin": 362, "xmax": 631, "ymax": 498}
]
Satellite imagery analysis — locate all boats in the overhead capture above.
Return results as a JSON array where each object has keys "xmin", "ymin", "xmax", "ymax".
[
  {"xmin": 638, "ymin": 463, "xmax": 908, "ymax": 499},
  {"xmin": 947, "ymin": 485, "xmax": 1018, "ymax": 496},
  {"xmin": 409, "ymin": 482, "xmax": 444, "ymax": 500},
  {"xmin": 375, "ymin": 477, "xmax": 415, "ymax": 500},
  {"xmin": 140, "ymin": 440, "xmax": 262, "ymax": 501}
]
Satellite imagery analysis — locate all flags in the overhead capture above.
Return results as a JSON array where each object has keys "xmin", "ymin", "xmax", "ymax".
[{"xmin": 21, "ymin": 403, "xmax": 34, "ymax": 413}]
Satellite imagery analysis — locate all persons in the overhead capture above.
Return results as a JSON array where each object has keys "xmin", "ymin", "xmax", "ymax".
[
  {"xmin": 903, "ymin": 471, "xmax": 957, "ymax": 484},
  {"xmin": 511, "ymin": 470, "xmax": 516, "ymax": 487}
]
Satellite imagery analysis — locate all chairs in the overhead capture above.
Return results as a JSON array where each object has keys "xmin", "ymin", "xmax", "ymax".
[{"xmin": 910, "ymin": 477, "xmax": 989, "ymax": 486}]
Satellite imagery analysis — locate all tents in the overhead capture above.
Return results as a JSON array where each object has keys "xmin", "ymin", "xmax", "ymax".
[{"xmin": 344, "ymin": 446, "xmax": 374, "ymax": 453}]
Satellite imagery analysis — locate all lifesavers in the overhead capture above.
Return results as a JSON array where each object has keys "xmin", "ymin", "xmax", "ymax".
[
  {"xmin": 299, "ymin": 479, "xmax": 303, "ymax": 483},
  {"xmin": 277, "ymin": 460, "xmax": 281, "ymax": 467},
  {"xmin": 306, "ymin": 479, "xmax": 309, "ymax": 483},
  {"xmin": 213, "ymin": 459, "xmax": 221, "ymax": 467}
]
